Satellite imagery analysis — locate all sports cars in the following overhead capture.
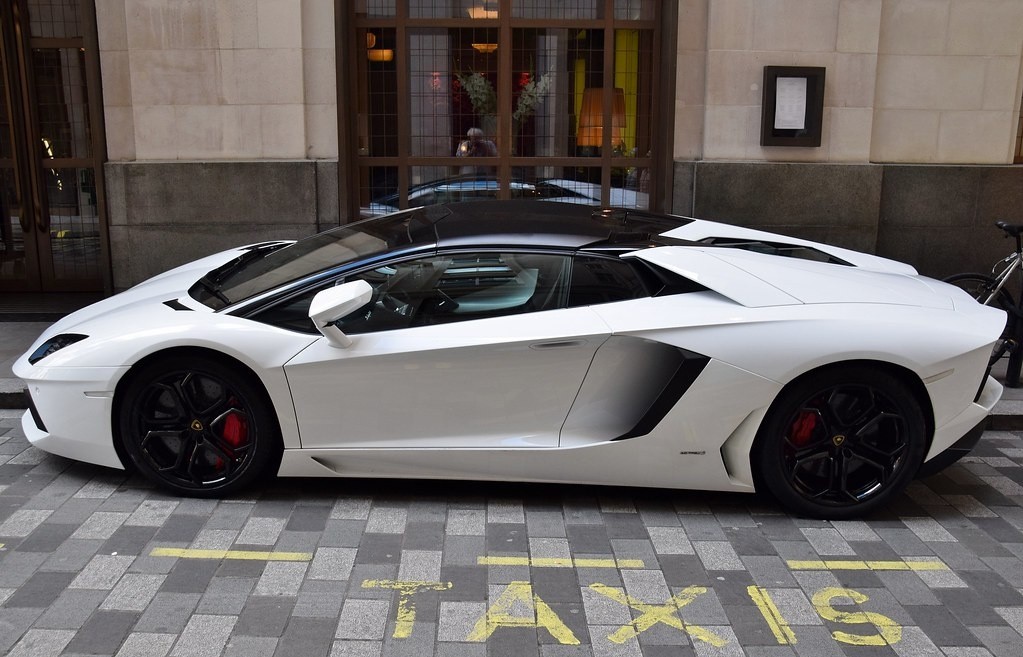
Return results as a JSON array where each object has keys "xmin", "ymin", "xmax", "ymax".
[
  {"xmin": 360, "ymin": 174, "xmax": 649, "ymax": 216},
  {"xmin": 11, "ymin": 200, "xmax": 1009, "ymax": 519}
]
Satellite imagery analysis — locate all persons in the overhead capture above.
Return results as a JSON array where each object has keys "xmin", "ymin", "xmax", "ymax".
[
  {"xmin": 456, "ymin": 128, "xmax": 496, "ymax": 174},
  {"xmin": 43, "ymin": 139, "xmax": 62, "ymax": 192}
]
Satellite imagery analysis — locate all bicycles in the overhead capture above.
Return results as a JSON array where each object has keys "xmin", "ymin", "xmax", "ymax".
[{"xmin": 943, "ymin": 219, "xmax": 1022, "ymax": 371}]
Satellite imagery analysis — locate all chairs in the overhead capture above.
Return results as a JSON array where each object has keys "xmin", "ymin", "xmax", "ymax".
[{"xmin": 523, "ymin": 258, "xmax": 563, "ymax": 312}]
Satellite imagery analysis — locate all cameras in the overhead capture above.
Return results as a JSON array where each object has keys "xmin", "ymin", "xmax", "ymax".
[{"xmin": 474, "ymin": 141, "xmax": 482, "ymax": 148}]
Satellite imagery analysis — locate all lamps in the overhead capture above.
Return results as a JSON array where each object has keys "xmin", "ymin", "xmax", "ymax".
[
  {"xmin": 577, "ymin": 88, "xmax": 627, "ymax": 177},
  {"xmin": 472, "ymin": 38, "xmax": 499, "ymax": 54}
]
